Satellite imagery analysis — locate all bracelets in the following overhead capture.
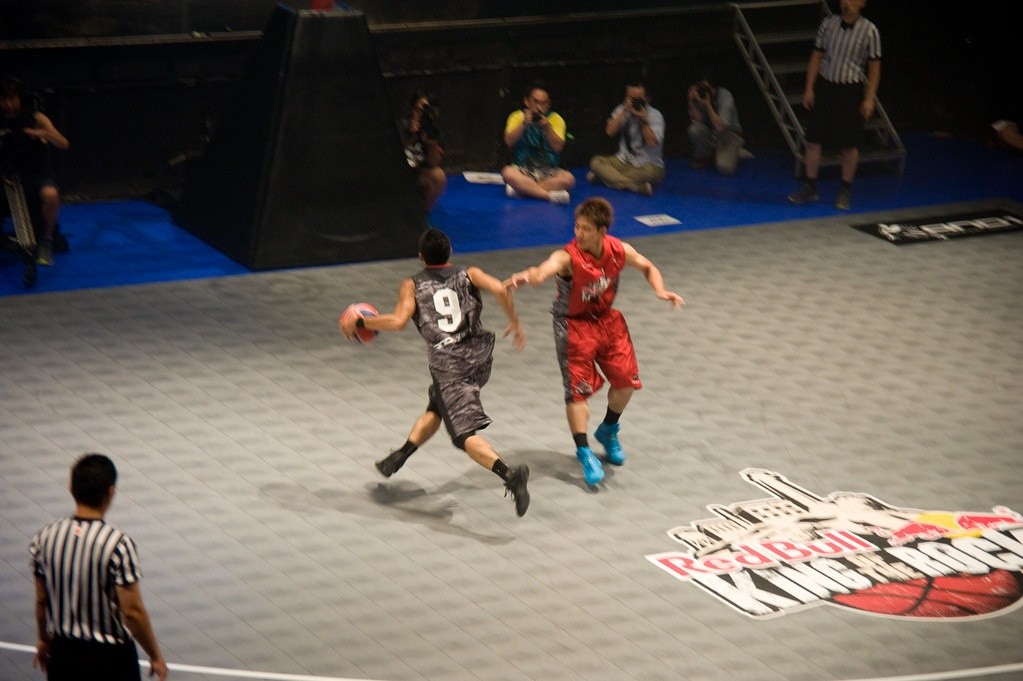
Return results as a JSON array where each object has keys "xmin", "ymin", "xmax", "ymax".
[{"xmin": 356, "ymin": 318, "xmax": 364, "ymax": 328}]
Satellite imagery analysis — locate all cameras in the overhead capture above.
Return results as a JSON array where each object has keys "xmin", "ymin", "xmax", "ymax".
[
  {"xmin": 415, "ymin": 104, "xmax": 440, "ymax": 141},
  {"xmin": 531, "ymin": 113, "xmax": 543, "ymax": 122},
  {"xmin": 631, "ymin": 97, "xmax": 646, "ymax": 112},
  {"xmin": 695, "ymin": 86, "xmax": 708, "ymax": 100}
]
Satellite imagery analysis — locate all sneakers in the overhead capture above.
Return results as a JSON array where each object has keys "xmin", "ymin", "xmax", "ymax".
[
  {"xmin": 34, "ymin": 240, "xmax": 55, "ymax": 267},
  {"xmin": 594, "ymin": 423, "xmax": 625, "ymax": 465},
  {"xmin": 788, "ymin": 183, "xmax": 819, "ymax": 203},
  {"xmin": 835, "ymin": 188, "xmax": 852, "ymax": 210},
  {"xmin": 575, "ymin": 446, "xmax": 605, "ymax": 486},
  {"xmin": 502, "ymin": 464, "xmax": 529, "ymax": 517},
  {"xmin": 376, "ymin": 448, "xmax": 406, "ymax": 477}
]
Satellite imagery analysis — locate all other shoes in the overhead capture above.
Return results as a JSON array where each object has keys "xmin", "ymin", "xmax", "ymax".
[
  {"xmin": 692, "ymin": 157, "xmax": 706, "ymax": 169},
  {"xmin": 640, "ymin": 181, "xmax": 653, "ymax": 196},
  {"xmin": 736, "ymin": 146, "xmax": 753, "ymax": 159},
  {"xmin": 586, "ymin": 171, "xmax": 602, "ymax": 183},
  {"xmin": 549, "ymin": 189, "xmax": 570, "ymax": 205},
  {"xmin": 506, "ymin": 183, "xmax": 518, "ymax": 198}
]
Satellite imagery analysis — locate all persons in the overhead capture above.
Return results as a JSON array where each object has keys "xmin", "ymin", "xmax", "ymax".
[
  {"xmin": 502, "ymin": 197, "xmax": 685, "ymax": 484},
  {"xmin": 30, "ymin": 454, "xmax": 169, "ymax": 680},
  {"xmin": 687, "ymin": 77, "xmax": 744, "ymax": 175},
  {"xmin": 340, "ymin": 229, "xmax": 530, "ymax": 517},
  {"xmin": 590, "ymin": 81, "xmax": 666, "ymax": 197},
  {"xmin": 394, "ymin": 91, "xmax": 447, "ymax": 214},
  {"xmin": 501, "ymin": 86, "xmax": 575, "ymax": 204},
  {"xmin": 0, "ymin": 78, "xmax": 70, "ymax": 266},
  {"xmin": 787, "ymin": 0, "xmax": 881, "ymax": 209}
]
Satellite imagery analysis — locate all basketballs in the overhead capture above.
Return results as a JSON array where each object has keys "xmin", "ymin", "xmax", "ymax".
[{"xmin": 340, "ymin": 303, "xmax": 381, "ymax": 345}]
